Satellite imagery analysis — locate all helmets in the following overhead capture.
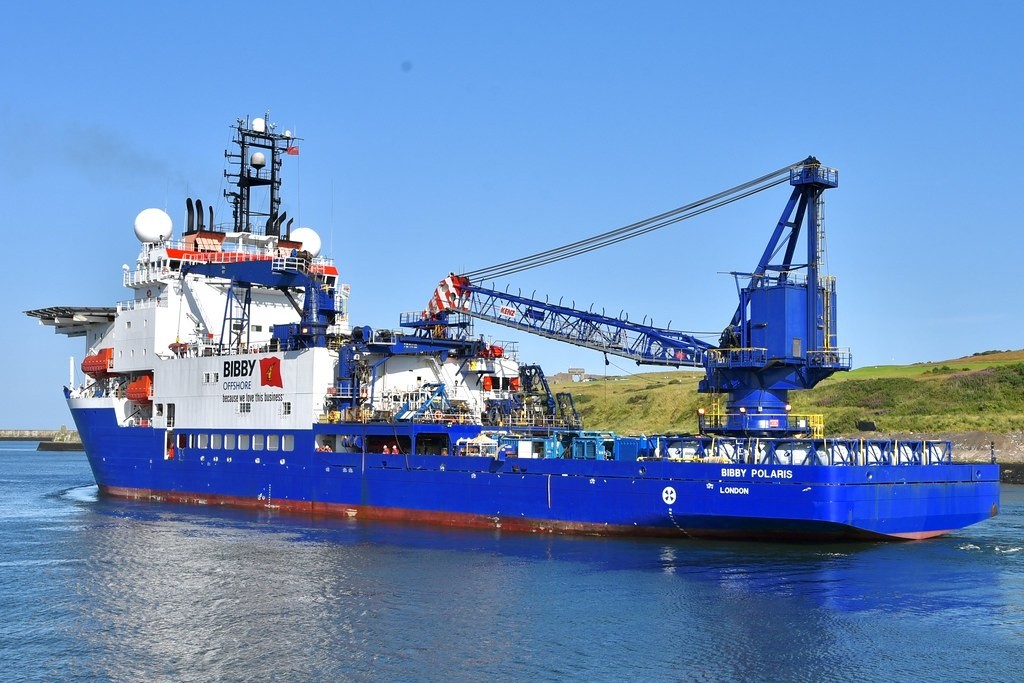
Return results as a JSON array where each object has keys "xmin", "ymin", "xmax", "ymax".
[
  {"xmin": 383, "ymin": 445, "xmax": 388, "ymax": 448},
  {"xmin": 392, "ymin": 445, "xmax": 397, "ymax": 449}
]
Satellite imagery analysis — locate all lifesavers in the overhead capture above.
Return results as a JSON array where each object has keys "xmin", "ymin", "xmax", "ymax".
[{"xmin": 433, "ymin": 410, "xmax": 443, "ymax": 420}]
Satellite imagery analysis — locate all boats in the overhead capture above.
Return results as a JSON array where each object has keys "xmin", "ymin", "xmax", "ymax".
[{"xmin": 22, "ymin": 115, "xmax": 1003, "ymax": 551}]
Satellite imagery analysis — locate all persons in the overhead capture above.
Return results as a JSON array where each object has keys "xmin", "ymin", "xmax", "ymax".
[
  {"xmin": 316, "ymin": 445, "xmax": 332, "ymax": 452},
  {"xmin": 392, "ymin": 446, "xmax": 399, "ymax": 454},
  {"xmin": 114, "ymin": 381, "xmax": 119, "ymax": 397},
  {"xmin": 382, "ymin": 445, "xmax": 390, "ymax": 454}
]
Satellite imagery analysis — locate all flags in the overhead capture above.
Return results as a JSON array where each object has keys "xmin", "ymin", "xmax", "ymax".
[{"xmin": 288, "ymin": 146, "xmax": 298, "ymax": 155}]
